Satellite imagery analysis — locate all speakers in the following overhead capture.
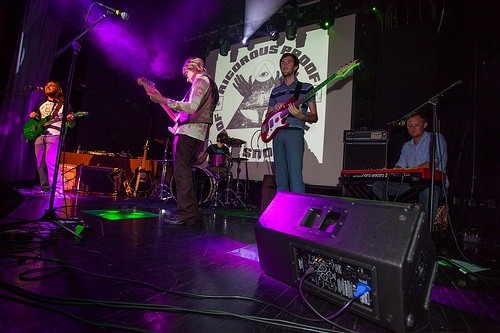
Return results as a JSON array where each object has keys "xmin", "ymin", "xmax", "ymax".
[
  {"xmin": 343, "ymin": 142, "xmax": 401, "ymax": 203},
  {"xmin": 253, "ymin": 189, "xmax": 438, "ymax": 333},
  {"xmin": 261, "ymin": 175, "xmax": 278, "ymax": 214},
  {"xmin": 0, "ymin": 179, "xmax": 25, "ymax": 219}
]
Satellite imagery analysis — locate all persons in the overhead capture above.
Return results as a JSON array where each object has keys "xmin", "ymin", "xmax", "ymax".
[
  {"xmin": 393, "ymin": 113, "xmax": 449, "ymax": 242},
  {"xmin": 27, "ymin": 81, "xmax": 75, "ymax": 198},
  {"xmin": 145, "ymin": 56, "xmax": 219, "ymax": 226},
  {"xmin": 265, "ymin": 53, "xmax": 318, "ymax": 194},
  {"xmin": 192, "ymin": 132, "xmax": 233, "ymax": 208}
]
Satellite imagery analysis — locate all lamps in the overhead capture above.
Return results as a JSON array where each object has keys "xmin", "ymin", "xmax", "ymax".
[{"xmin": 219, "ymin": 14, "xmax": 336, "ymax": 56}]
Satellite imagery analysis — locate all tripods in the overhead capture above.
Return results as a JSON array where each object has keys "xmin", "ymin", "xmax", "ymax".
[
  {"xmin": 0, "ymin": 11, "xmax": 113, "ymax": 243},
  {"xmin": 150, "ymin": 139, "xmax": 172, "ymax": 201},
  {"xmin": 204, "ymin": 141, "xmax": 255, "ymax": 212}
]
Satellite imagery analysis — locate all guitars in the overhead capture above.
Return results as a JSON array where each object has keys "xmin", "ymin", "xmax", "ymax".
[
  {"xmin": 128, "ymin": 140, "xmax": 156, "ymax": 193},
  {"xmin": 137, "ymin": 76, "xmax": 181, "ymax": 134},
  {"xmin": 260, "ymin": 59, "xmax": 362, "ymax": 143},
  {"xmin": 24, "ymin": 111, "xmax": 89, "ymax": 141}
]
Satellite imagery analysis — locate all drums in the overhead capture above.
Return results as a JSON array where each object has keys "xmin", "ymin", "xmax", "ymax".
[
  {"xmin": 155, "ymin": 160, "xmax": 173, "ymax": 184},
  {"xmin": 208, "ymin": 152, "xmax": 230, "ymax": 173},
  {"xmin": 169, "ymin": 165, "xmax": 218, "ymax": 207}
]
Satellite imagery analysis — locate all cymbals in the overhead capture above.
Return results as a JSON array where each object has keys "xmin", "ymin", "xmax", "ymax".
[
  {"xmin": 226, "ymin": 157, "xmax": 249, "ymax": 162},
  {"xmin": 221, "ymin": 138, "xmax": 245, "ymax": 146}
]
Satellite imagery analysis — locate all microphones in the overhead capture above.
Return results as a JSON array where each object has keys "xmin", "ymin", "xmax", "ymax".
[
  {"xmin": 391, "ymin": 121, "xmax": 405, "ymax": 126},
  {"xmin": 28, "ymin": 86, "xmax": 43, "ymax": 90},
  {"xmin": 98, "ymin": 3, "xmax": 129, "ymax": 20}
]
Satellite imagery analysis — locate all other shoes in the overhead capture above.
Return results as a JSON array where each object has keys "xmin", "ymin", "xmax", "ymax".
[
  {"xmin": 164, "ymin": 214, "xmax": 186, "ymax": 224},
  {"xmin": 40, "ymin": 187, "xmax": 49, "ymax": 194}
]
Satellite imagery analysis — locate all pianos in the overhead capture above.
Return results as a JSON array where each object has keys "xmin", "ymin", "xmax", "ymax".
[{"xmin": 336, "ymin": 165, "xmax": 448, "ymax": 204}]
